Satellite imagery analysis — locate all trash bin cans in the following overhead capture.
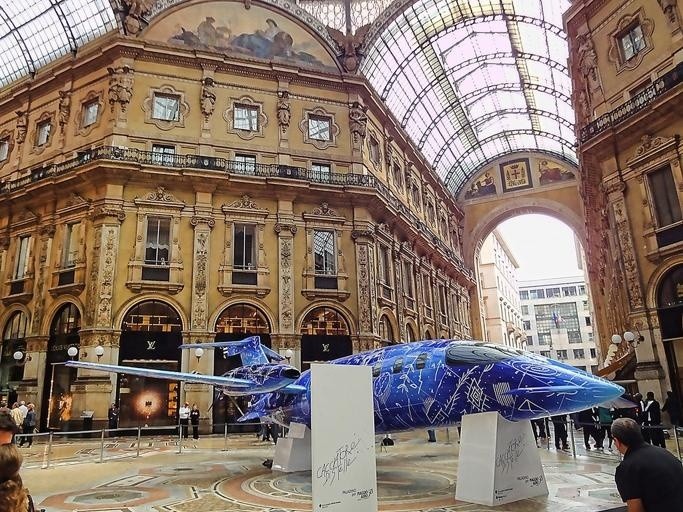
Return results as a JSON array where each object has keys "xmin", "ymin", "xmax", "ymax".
[{"xmin": 83, "ymin": 417, "xmax": 92, "ymax": 438}]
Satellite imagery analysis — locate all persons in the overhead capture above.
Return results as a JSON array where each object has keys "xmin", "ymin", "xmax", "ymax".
[
  {"xmin": 178, "ymin": 401, "xmax": 189, "ymax": 438},
  {"xmin": 610, "ymin": 418, "xmax": 682, "ymax": 512},
  {"xmin": 111, "ymin": 403, "xmax": 119, "ymax": 435},
  {"xmin": 0, "ymin": 399, "xmax": 35, "ymax": 448},
  {"xmin": 531, "ymin": 391, "xmax": 665, "ymax": 452},
  {"xmin": 190, "ymin": 403, "xmax": 199, "ymax": 438},
  {"xmin": 0, "ymin": 443, "xmax": 35, "ymax": 512}
]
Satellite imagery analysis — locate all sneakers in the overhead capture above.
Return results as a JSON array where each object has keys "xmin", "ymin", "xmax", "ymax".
[
  {"xmin": 556, "ymin": 443, "xmax": 570, "ymax": 449},
  {"xmin": 585, "ymin": 441, "xmax": 613, "ymax": 451}
]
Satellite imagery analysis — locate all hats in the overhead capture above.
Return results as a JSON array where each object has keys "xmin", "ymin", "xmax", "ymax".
[
  {"xmin": 21, "ymin": 401, "xmax": 25, "ymax": 405},
  {"xmin": 26, "ymin": 404, "xmax": 35, "ymax": 408}
]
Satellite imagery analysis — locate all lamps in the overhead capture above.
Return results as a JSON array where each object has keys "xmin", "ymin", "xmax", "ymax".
[
  {"xmin": 602, "ymin": 334, "xmax": 621, "ymax": 368},
  {"xmin": 623, "ymin": 331, "xmax": 644, "ymax": 349},
  {"xmin": 66, "ymin": 345, "xmax": 106, "ymax": 362},
  {"xmin": 284, "ymin": 348, "xmax": 293, "ymax": 363},
  {"xmin": 12, "ymin": 350, "xmax": 32, "ymax": 366},
  {"xmin": 194, "ymin": 346, "xmax": 204, "ymax": 364}
]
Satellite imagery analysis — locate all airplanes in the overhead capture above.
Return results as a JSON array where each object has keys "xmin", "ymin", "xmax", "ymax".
[{"xmin": 52, "ymin": 331, "xmax": 639, "ymax": 448}]
